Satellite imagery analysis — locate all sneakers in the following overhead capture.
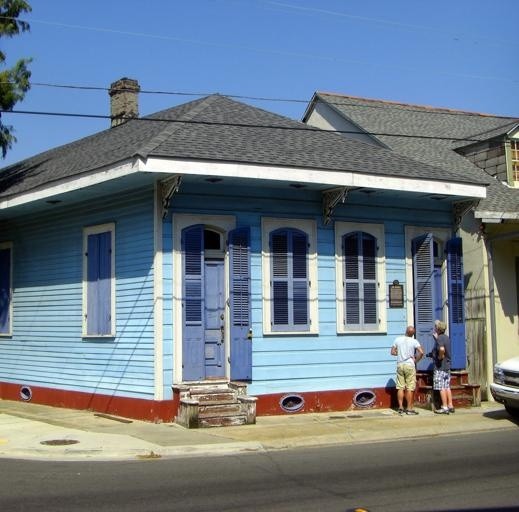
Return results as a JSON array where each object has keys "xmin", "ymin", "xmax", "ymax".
[
  {"xmin": 434, "ymin": 407, "xmax": 455, "ymax": 415},
  {"xmin": 398, "ymin": 409, "xmax": 419, "ymax": 416}
]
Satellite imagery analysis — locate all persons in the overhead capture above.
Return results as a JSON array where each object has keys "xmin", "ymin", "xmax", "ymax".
[{"xmin": 390, "ymin": 319, "xmax": 455, "ymax": 415}]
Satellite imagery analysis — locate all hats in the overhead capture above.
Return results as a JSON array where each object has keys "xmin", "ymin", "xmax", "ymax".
[{"xmin": 434, "ymin": 319, "xmax": 447, "ymax": 331}]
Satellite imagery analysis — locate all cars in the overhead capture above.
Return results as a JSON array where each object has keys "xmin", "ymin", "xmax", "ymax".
[{"xmin": 490, "ymin": 356, "xmax": 519, "ymax": 415}]
